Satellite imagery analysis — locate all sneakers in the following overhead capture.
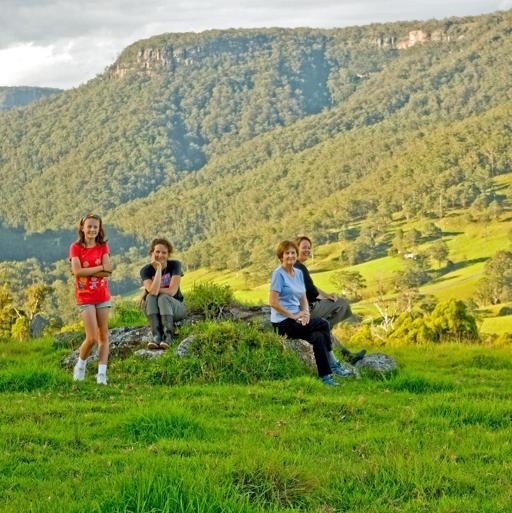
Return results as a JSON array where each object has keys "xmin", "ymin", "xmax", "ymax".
[
  {"xmin": 330, "ymin": 359, "xmax": 350, "ymax": 377},
  {"xmin": 350, "ymin": 349, "xmax": 366, "ymax": 364},
  {"xmin": 97, "ymin": 373, "xmax": 107, "ymax": 385},
  {"xmin": 321, "ymin": 373, "xmax": 341, "ymax": 386},
  {"xmin": 73, "ymin": 362, "xmax": 86, "ymax": 381}
]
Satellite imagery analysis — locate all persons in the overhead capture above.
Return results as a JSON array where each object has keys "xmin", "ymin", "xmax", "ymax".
[
  {"xmin": 267, "ymin": 240, "xmax": 351, "ymax": 387},
  {"xmin": 135, "ymin": 239, "xmax": 187, "ymax": 348},
  {"xmin": 293, "ymin": 236, "xmax": 367, "ymax": 364},
  {"xmin": 68, "ymin": 211, "xmax": 113, "ymax": 387}
]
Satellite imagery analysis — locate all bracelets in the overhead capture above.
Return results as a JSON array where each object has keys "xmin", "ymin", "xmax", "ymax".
[{"xmin": 101, "ymin": 265, "xmax": 105, "ymax": 271}]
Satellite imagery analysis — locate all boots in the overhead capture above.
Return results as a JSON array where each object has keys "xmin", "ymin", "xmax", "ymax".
[
  {"xmin": 160, "ymin": 315, "xmax": 174, "ymax": 349},
  {"xmin": 147, "ymin": 313, "xmax": 161, "ymax": 348}
]
